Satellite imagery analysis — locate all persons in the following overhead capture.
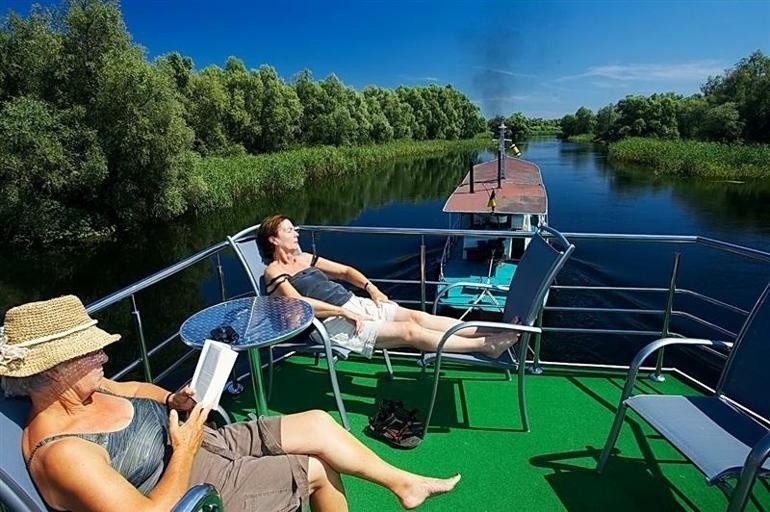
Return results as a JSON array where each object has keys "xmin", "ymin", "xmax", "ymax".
[
  {"xmin": 0, "ymin": 292, "xmax": 463, "ymax": 511},
  {"xmin": 480, "ymin": 216, "xmax": 498, "ymax": 231},
  {"xmin": 256, "ymin": 215, "xmax": 524, "ymax": 360}
]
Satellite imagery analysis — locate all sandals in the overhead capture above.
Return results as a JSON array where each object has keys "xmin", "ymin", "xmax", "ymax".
[{"xmin": 367, "ymin": 399, "xmax": 425, "ymax": 450}]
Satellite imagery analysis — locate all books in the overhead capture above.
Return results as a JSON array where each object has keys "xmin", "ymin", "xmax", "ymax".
[{"xmin": 186, "ymin": 339, "xmax": 238, "ymax": 411}]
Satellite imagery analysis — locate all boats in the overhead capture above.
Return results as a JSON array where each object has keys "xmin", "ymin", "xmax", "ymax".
[{"xmin": 435, "ymin": 116, "xmax": 552, "ymax": 338}]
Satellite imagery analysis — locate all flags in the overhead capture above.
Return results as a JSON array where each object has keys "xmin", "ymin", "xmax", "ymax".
[{"xmin": 487, "ymin": 191, "xmax": 497, "ymax": 207}]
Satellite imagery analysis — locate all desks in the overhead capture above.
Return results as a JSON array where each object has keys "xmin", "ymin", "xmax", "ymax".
[{"xmin": 180, "ymin": 295, "xmax": 315, "ymax": 419}]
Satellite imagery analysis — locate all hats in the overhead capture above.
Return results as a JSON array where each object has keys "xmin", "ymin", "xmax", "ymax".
[{"xmin": 1, "ymin": 295, "xmax": 122, "ymax": 377}]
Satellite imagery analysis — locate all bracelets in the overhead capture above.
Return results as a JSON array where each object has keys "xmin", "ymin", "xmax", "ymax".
[
  {"xmin": 364, "ymin": 281, "xmax": 372, "ymax": 290},
  {"xmin": 165, "ymin": 392, "xmax": 185, "ymax": 417}
]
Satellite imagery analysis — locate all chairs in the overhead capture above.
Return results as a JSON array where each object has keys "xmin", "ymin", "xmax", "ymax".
[
  {"xmin": 229, "ymin": 223, "xmax": 396, "ymax": 433},
  {"xmin": 596, "ymin": 284, "xmax": 770, "ymax": 510},
  {"xmin": 0, "ymin": 386, "xmax": 219, "ymax": 512},
  {"xmin": 415, "ymin": 222, "xmax": 576, "ymax": 432}
]
